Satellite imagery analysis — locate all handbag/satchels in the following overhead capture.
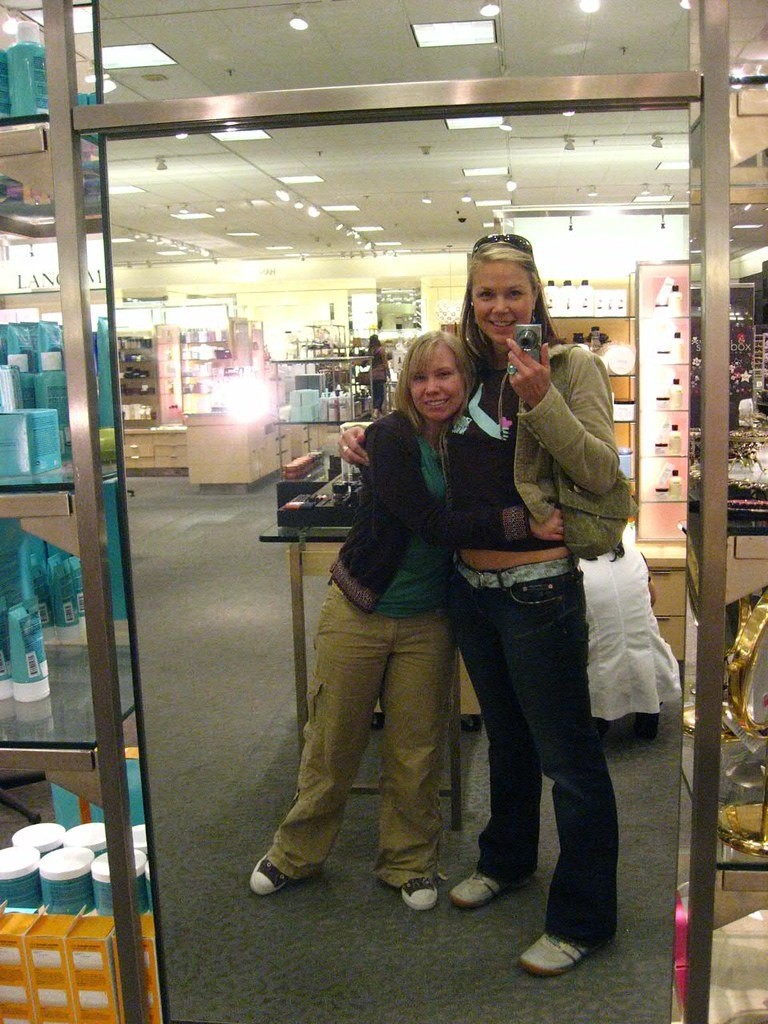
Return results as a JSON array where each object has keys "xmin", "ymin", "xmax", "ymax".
[{"xmin": 547, "ymin": 343, "xmax": 632, "ymax": 560}]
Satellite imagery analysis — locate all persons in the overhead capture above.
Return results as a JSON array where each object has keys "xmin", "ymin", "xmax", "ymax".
[
  {"xmin": 251, "ymin": 330, "xmax": 565, "ymax": 910},
  {"xmin": 338, "ymin": 234, "xmax": 620, "ymax": 978},
  {"xmin": 355, "ymin": 334, "xmax": 391, "ymax": 422},
  {"xmin": 576, "ymin": 526, "xmax": 682, "ymax": 749}
]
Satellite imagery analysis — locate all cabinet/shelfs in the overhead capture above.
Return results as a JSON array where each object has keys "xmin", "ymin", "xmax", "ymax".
[
  {"xmin": 116, "ymin": 311, "xmax": 183, "ymax": 422},
  {"xmin": 523, "ymin": 274, "xmax": 642, "ymax": 539},
  {"xmin": 636, "ymin": 256, "xmax": 690, "ymax": 544},
  {"xmin": 667, "ymin": 0, "xmax": 768, "ymax": 1023},
  {"xmin": 0, "ymin": 10, "xmax": 165, "ymax": 1023},
  {"xmin": 174, "ymin": 318, "xmax": 275, "ymax": 424}
]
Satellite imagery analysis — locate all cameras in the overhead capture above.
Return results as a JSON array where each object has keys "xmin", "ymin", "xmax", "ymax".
[{"xmin": 515, "ymin": 323, "xmax": 542, "ymax": 365}]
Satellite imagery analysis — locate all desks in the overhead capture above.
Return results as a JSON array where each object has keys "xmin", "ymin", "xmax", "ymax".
[{"xmin": 258, "ymin": 462, "xmax": 468, "ymax": 830}]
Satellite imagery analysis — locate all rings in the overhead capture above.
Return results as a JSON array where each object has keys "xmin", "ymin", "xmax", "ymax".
[
  {"xmin": 507, "ymin": 364, "xmax": 517, "ymax": 375},
  {"xmin": 341, "ymin": 446, "xmax": 348, "ymax": 452}
]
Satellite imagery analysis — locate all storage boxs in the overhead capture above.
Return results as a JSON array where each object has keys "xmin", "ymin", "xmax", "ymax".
[{"xmin": 0, "ymin": 900, "xmax": 163, "ymax": 1024}]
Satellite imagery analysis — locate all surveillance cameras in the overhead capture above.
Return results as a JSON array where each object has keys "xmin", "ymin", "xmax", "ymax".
[{"xmin": 458, "ymin": 217, "xmax": 466, "ymax": 223}]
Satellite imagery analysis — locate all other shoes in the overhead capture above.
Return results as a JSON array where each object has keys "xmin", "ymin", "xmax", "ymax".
[{"xmin": 633, "ymin": 713, "xmax": 660, "ymax": 740}]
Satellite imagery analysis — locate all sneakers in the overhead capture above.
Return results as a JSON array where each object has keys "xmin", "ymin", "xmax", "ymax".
[
  {"xmin": 400, "ymin": 876, "xmax": 436, "ymax": 909},
  {"xmin": 517, "ymin": 933, "xmax": 598, "ymax": 976},
  {"xmin": 449, "ymin": 870, "xmax": 529, "ymax": 906},
  {"xmin": 249, "ymin": 856, "xmax": 292, "ymax": 896}
]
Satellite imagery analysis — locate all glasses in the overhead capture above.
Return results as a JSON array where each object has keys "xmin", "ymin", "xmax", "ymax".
[{"xmin": 470, "ymin": 233, "xmax": 535, "ymax": 257}]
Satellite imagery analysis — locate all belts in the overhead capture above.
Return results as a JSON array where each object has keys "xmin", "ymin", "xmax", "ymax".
[{"xmin": 452, "ymin": 551, "xmax": 580, "ymax": 588}]
